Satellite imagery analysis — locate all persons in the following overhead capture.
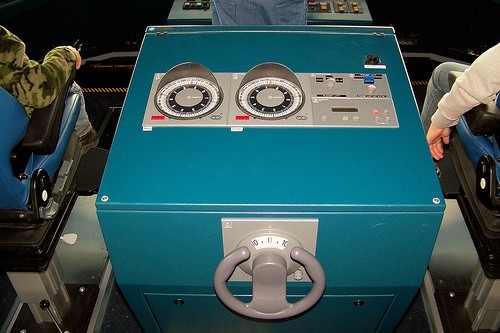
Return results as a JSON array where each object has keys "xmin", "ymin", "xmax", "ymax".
[
  {"xmin": 421, "ymin": 42, "xmax": 500, "ymax": 161},
  {"xmin": 0, "ymin": 24, "xmax": 99, "ymax": 155},
  {"xmin": 210, "ymin": 0, "xmax": 308, "ymax": 26}
]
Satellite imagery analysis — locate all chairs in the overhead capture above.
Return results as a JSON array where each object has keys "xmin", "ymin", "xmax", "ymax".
[
  {"xmin": 450, "ymin": 93, "xmax": 500, "ymax": 279},
  {"xmin": 0, "ymin": 76, "xmax": 82, "ymax": 276}
]
